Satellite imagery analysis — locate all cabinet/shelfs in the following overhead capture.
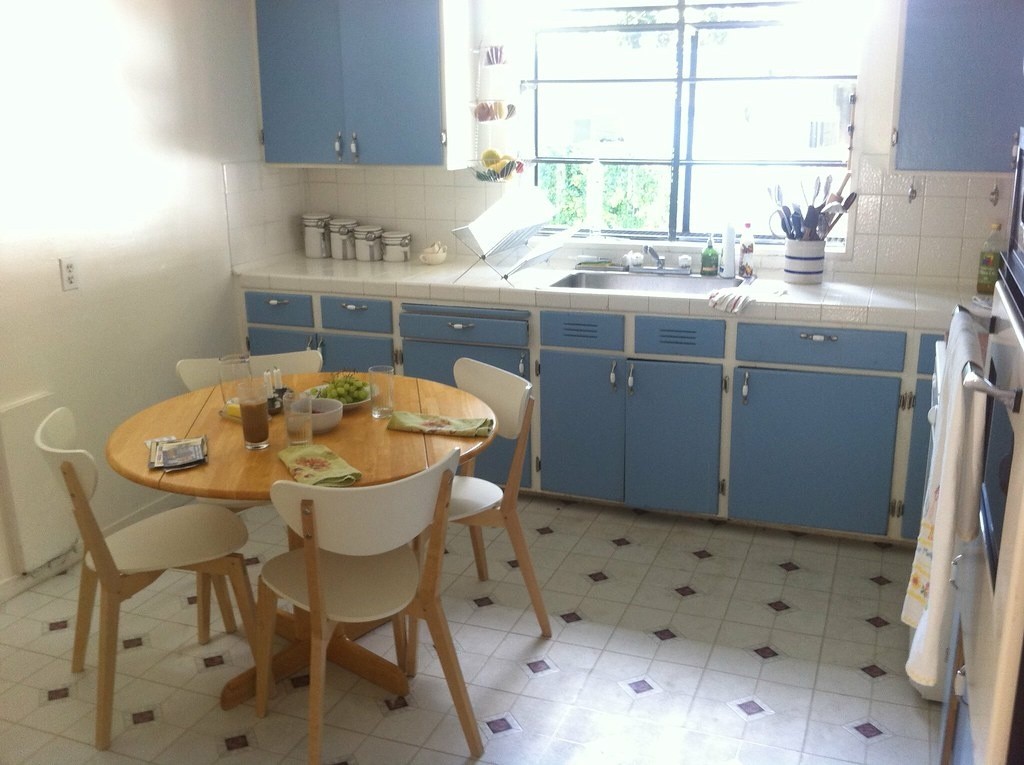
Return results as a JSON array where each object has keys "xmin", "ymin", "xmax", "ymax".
[
  {"xmin": 893, "ymin": 327, "xmax": 945, "ymax": 543},
  {"xmin": 892, "ymin": 1, "xmax": 1024, "ymax": 173},
  {"xmin": 536, "ymin": 312, "xmax": 726, "ymax": 516},
  {"xmin": 254, "ymin": 0, "xmax": 477, "ymax": 169},
  {"xmin": 724, "ymin": 320, "xmax": 910, "ymax": 537},
  {"xmin": 397, "ymin": 311, "xmax": 531, "ymax": 491},
  {"xmin": 246, "ymin": 293, "xmax": 394, "ymax": 380}
]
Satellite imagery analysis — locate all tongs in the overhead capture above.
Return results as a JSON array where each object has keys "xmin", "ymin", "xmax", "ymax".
[{"xmin": 811, "ymin": 175, "xmax": 833, "ymax": 206}]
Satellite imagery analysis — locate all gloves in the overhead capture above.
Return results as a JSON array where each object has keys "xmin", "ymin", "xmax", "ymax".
[{"xmin": 708, "ymin": 278, "xmax": 791, "ymax": 314}]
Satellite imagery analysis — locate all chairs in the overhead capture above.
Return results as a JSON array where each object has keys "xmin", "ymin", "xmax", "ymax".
[
  {"xmin": 252, "ymin": 447, "xmax": 486, "ymax": 765},
  {"xmin": 34, "ymin": 405, "xmax": 253, "ymax": 746},
  {"xmin": 171, "ymin": 349, "xmax": 323, "ymax": 639},
  {"xmin": 397, "ymin": 355, "xmax": 552, "ymax": 676}
]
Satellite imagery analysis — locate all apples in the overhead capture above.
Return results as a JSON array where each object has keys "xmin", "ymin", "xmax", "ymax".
[
  {"xmin": 473, "ymin": 101, "xmax": 508, "ymax": 121},
  {"xmin": 482, "ymin": 149, "xmax": 516, "ymax": 174}
]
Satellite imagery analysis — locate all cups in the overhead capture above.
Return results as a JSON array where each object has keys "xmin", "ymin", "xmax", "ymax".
[
  {"xmin": 218, "ymin": 353, "xmax": 251, "ymax": 417},
  {"xmin": 368, "ymin": 365, "xmax": 395, "ymax": 418},
  {"xmin": 282, "ymin": 392, "xmax": 313, "ymax": 447},
  {"xmin": 784, "ymin": 237, "xmax": 826, "ymax": 284},
  {"xmin": 239, "ymin": 383, "xmax": 270, "ymax": 449},
  {"xmin": 419, "ymin": 242, "xmax": 448, "ymax": 264}
]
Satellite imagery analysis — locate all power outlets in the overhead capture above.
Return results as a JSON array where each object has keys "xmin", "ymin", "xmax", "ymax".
[{"xmin": 58, "ymin": 258, "xmax": 82, "ymax": 290}]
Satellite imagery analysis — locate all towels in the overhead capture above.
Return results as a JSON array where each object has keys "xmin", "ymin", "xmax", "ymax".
[{"xmin": 900, "ymin": 311, "xmax": 987, "ymax": 703}]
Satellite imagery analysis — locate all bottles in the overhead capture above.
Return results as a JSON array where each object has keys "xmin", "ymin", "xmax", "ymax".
[
  {"xmin": 976, "ymin": 223, "xmax": 1001, "ymax": 294},
  {"xmin": 301, "ymin": 213, "xmax": 411, "ymax": 261},
  {"xmin": 718, "ymin": 222, "xmax": 754, "ymax": 279}
]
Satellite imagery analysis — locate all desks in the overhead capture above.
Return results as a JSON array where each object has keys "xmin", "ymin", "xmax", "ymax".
[{"xmin": 106, "ymin": 374, "xmax": 494, "ymax": 707}]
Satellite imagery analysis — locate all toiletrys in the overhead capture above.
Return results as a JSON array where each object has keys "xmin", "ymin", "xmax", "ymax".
[{"xmin": 700, "ymin": 230, "xmax": 719, "ymax": 276}]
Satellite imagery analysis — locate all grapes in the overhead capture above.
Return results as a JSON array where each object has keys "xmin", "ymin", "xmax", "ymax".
[{"xmin": 309, "ymin": 374, "xmax": 369, "ymax": 403}]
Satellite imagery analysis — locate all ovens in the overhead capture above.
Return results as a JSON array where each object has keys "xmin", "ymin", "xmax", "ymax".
[{"xmin": 938, "ymin": 279, "xmax": 1024, "ymax": 765}]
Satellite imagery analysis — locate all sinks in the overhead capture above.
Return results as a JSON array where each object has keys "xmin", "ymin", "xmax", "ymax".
[{"xmin": 549, "ymin": 271, "xmax": 757, "ymax": 294}]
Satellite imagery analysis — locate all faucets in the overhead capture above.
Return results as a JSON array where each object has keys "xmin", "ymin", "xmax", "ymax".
[{"xmin": 629, "ymin": 245, "xmax": 692, "ymax": 275}]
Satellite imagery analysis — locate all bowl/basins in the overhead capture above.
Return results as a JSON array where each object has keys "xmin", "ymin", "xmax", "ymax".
[
  {"xmin": 299, "ymin": 383, "xmax": 379, "ymax": 410},
  {"xmin": 290, "ymin": 398, "xmax": 343, "ymax": 434}
]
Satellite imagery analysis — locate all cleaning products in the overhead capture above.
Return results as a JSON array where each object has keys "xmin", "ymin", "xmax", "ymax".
[
  {"xmin": 738, "ymin": 222, "xmax": 756, "ymax": 278},
  {"xmin": 718, "ymin": 221, "xmax": 736, "ymax": 279}
]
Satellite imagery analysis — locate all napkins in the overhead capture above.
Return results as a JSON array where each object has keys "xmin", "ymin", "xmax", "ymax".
[
  {"xmin": 279, "ymin": 445, "xmax": 362, "ymax": 494},
  {"xmin": 386, "ymin": 411, "xmax": 494, "ymax": 439}
]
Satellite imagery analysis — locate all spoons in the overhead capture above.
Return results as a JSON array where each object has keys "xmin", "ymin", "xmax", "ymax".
[{"xmin": 776, "ymin": 186, "xmax": 791, "ymax": 234}]
